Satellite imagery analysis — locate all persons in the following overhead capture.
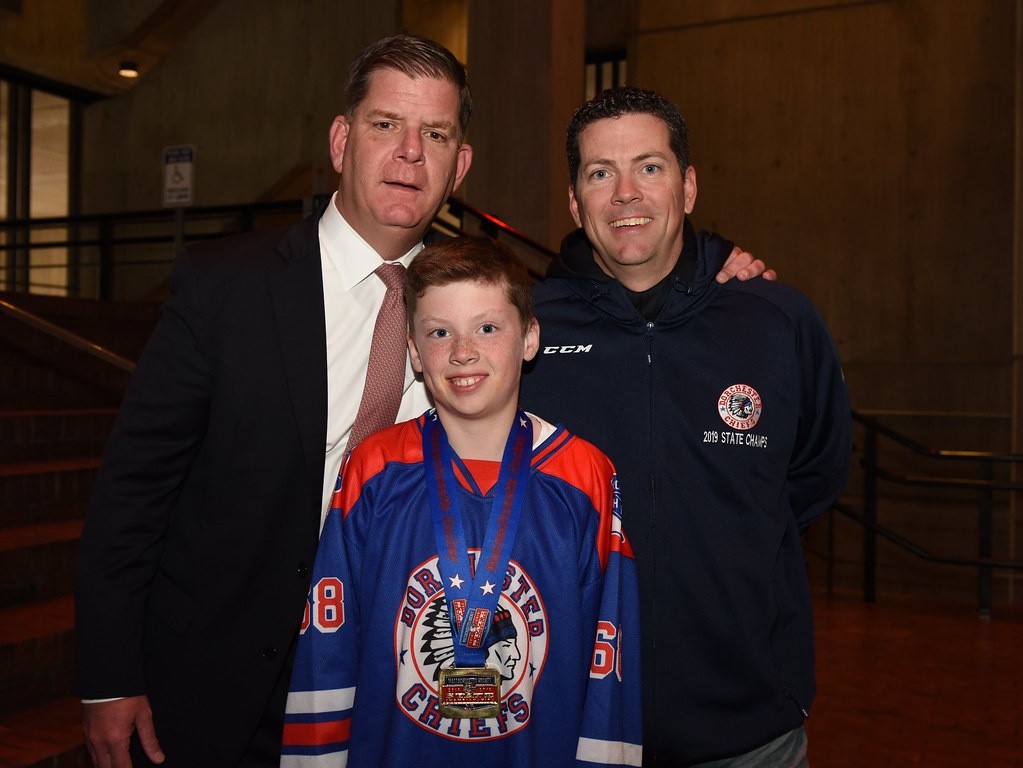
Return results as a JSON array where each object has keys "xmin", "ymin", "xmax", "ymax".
[
  {"xmin": 517, "ymin": 88, "xmax": 849, "ymax": 768},
  {"xmin": 76, "ymin": 34, "xmax": 781, "ymax": 768},
  {"xmin": 278, "ymin": 236, "xmax": 644, "ymax": 768}
]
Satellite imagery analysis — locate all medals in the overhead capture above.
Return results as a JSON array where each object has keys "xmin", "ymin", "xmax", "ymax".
[{"xmin": 436, "ymin": 668, "xmax": 501, "ymax": 718}]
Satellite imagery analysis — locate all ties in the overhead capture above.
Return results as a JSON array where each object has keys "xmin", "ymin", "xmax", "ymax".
[{"xmin": 345, "ymin": 263, "xmax": 411, "ymax": 456}]
